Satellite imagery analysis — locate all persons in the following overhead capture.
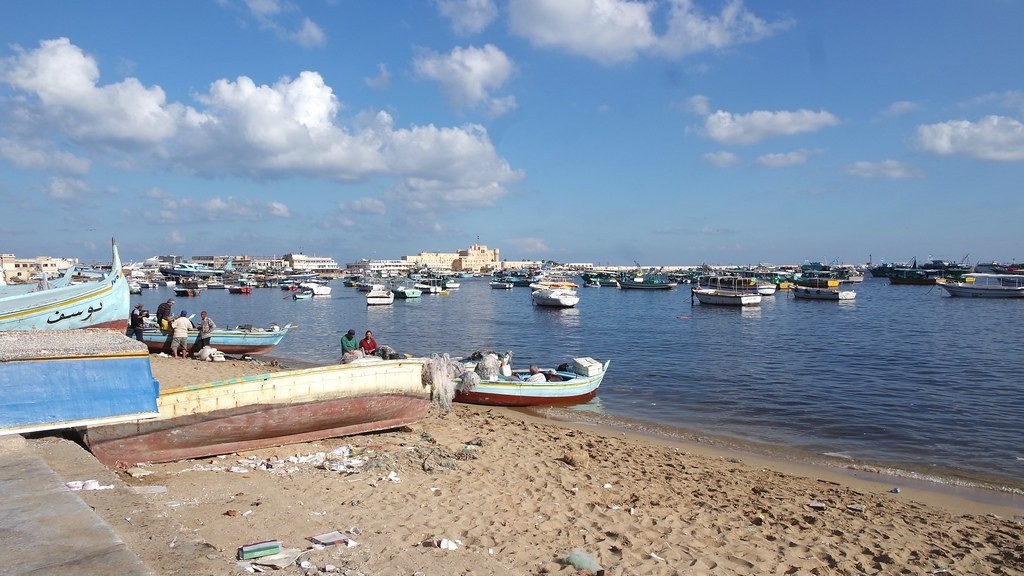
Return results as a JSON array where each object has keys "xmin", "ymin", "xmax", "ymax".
[
  {"xmin": 170, "ymin": 310, "xmax": 193, "ymax": 359},
  {"xmin": 341, "ymin": 329, "xmax": 358, "ymax": 355},
  {"xmin": 359, "ymin": 330, "xmax": 377, "ymax": 355},
  {"xmin": 515, "ymin": 364, "xmax": 564, "ymax": 382},
  {"xmin": 157, "ymin": 298, "xmax": 175, "ymax": 329},
  {"xmin": 198, "ymin": 311, "xmax": 217, "ymax": 349},
  {"xmin": 131, "ymin": 302, "xmax": 146, "ymax": 342}
]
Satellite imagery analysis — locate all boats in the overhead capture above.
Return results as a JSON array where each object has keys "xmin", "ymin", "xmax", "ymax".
[
  {"xmin": 124, "ymin": 322, "xmax": 294, "ymax": 355},
  {"xmin": 532, "ymin": 291, "xmax": 585, "ymax": 309},
  {"xmin": 1, "ymin": 261, "xmax": 953, "ymax": 307},
  {"xmin": 0, "ymin": 235, "xmax": 132, "ymax": 336},
  {"xmin": 692, "ymin": 289, "xmax": 764, "ymax": 306},
  {"xmin": 791, "ymin": 289, "xmax": 856, "ymax": 300},
  {"xmin": 341, "ymin": 350, "xmax": 612, "ymax": 407},
  {"xmin": 938, "ymin": 273, "xmax": 1024, "ymax": 296}
]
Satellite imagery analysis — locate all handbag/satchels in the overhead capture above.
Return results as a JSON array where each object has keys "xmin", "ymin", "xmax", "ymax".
[{"xmin": 161, "ymin": 319, "xmax": 174, "ymax": 331}]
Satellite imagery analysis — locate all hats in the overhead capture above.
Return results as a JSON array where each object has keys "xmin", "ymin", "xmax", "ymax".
[
  {"xmin": 134, "ymin": 302, "xmax": 144, "ymax": 308},
  {"xmin": 348, "ymin": 329, "xmax": 355, "ymax": 337},
  {"xmin": 168, "ymin": 298, "xmax": 175, "ymax": 303},
  {"xmin": 181, "ymin": 310, "xmax": 187, "ymax": 314}
]
